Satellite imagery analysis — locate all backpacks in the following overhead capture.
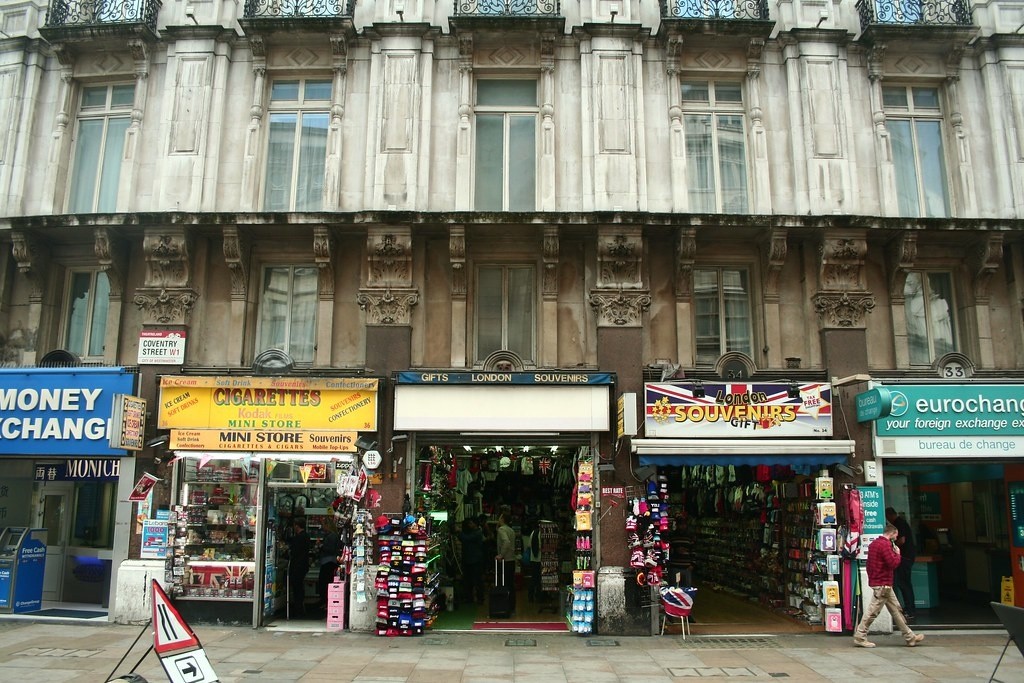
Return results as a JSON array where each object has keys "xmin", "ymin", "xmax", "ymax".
[
  {"xmin": 521, "ymin": 456, "xmax": 534, "ymax": 475},
  {"xmin": 539, "ymin": 457, "xmax": 552, "ymax": 475}
]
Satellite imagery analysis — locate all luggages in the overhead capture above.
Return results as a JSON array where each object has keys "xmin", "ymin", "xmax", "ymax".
[{"xmin": 489, "ymin": 558, "xmax": 511, "ymax": 619}]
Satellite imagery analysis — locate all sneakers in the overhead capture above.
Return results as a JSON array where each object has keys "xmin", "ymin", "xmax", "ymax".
[
  {"xmin": 903, "ymin": 608, "xmax": 917, "ymax": 619},
  {"xmin": 906, "ymin": 634, "xmax": 924, "ymax": 646},
  {"xmin": 854, "ymin": 638, "xmax": 876, "ymax": 648}
]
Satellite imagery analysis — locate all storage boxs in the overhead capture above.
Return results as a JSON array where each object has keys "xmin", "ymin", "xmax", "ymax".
[{"xmin": 327, "ymin": 580, "xmax": 348, "ymax": 630}]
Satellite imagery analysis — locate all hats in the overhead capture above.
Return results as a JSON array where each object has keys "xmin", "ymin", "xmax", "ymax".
[
  {"xmin": 374, "ymin": 516, "xmax": 426, "ymax": 637},
  {"xmin": 499, "ymin": 457, "xmax": 511, "ymax": 468},
  {"xmin": 625, "ymin": 481, "xmax": 669, "ymax": 587}
]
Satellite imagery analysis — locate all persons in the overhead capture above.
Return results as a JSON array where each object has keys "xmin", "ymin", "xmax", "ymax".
[
  {"xmin": 885, "ymin": 507, "xmax": 918, "ymax": 623},
  {"xmin": 496, "ymin": 511, "xmax": 516, "ymax": 615},
  {"xmin": 313, "ymin": 517, "xmax": 341, "ymax": 620},
  {"xmin": 282, "ymin": 518, "xmax": 310, "ymax": 619},
  {"xmin": 853, "ymin": 524, "xmax": 924, "ymax": 647},
  {"xmin": 455, "ymin": 514, "xmax": 494, "ymax": 603},
  {"xmin": 669, "ymin": 516, "xmax": 696, "ymax": 623},
  {"xmin": 528, "ymin": 523, "xmax": 541, "ymax": 601}
]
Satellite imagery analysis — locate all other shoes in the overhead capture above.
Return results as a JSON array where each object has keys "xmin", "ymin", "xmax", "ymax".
[
  {"xmin": 685, "ymin": 614, "xmax": 696, "ymax": 623},
  {"xmin": 666, "ymin": 614, "xmax": 675, "ymax": 622},
  {"xmin": 477, "ymin": 599, "xmax": 483, "ymax": 605},
  {"xmin": 508, "ymin": 608, "xmax": 516, "ymax": 613}
]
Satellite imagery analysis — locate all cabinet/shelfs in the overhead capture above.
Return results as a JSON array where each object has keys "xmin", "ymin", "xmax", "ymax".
[
  {"xmin": 174, "ymin": 475, "xmax": 347, "ymax": 578},
  {"xmin": 665, "ymin": 482, "xmax": 837, "ymax": 626},
  {"xmin": 537, "ymin": 520, "xmax": 560, "ymax": 613},
  {"xmin": 483, "ymin": 517, "xmax": 523, "ymax": 564},
  {"xmin": 426, "ymin": 541, "xmax": 441, "ymax": 626},
  {"xmin": 171, "ymin": 462, "xmax": 258, "ymax": 564}
]
